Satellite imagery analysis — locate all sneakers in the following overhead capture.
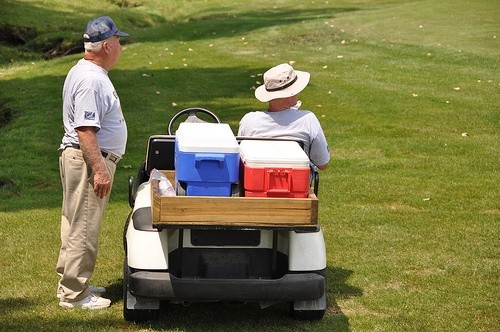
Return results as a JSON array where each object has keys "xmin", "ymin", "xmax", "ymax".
[
  {"xmin": 57, "ymin": 283, "xmax": 106, "ymax": 299},
  {"xmin": 59, "ymin": 292, "xmax": 111, "ymax": 309}
]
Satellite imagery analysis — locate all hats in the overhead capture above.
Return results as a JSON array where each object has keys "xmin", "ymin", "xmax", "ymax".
[
  {"xmin": 83, "ymin": 15, "xmax": 130, "ymax": 42},
  {"xmin": 254, "ymin": 63, "xmax": 311, "ymax": 102}
]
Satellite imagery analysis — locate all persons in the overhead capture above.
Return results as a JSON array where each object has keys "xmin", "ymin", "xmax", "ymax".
[
  {"xmin": 237, "ymin": 61, "xmax": 332, "ymax": 171},
  {"xmin": 56, "ymin": 16, "xmax": 128, "ymax": 309}
]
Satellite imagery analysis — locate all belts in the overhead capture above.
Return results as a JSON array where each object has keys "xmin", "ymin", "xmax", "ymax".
[{"xmin": 72, "ymin": 142, "xmax": 120, "ymax": 167}]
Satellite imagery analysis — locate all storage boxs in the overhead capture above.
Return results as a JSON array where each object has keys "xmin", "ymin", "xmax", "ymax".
[
  {"xmin": 174, "ymin": 122, "xmax": 239, "ymax": 197},
  {"xmin": 239, "ymin": 139, "xmax": 311, "ymax": 197}
]
